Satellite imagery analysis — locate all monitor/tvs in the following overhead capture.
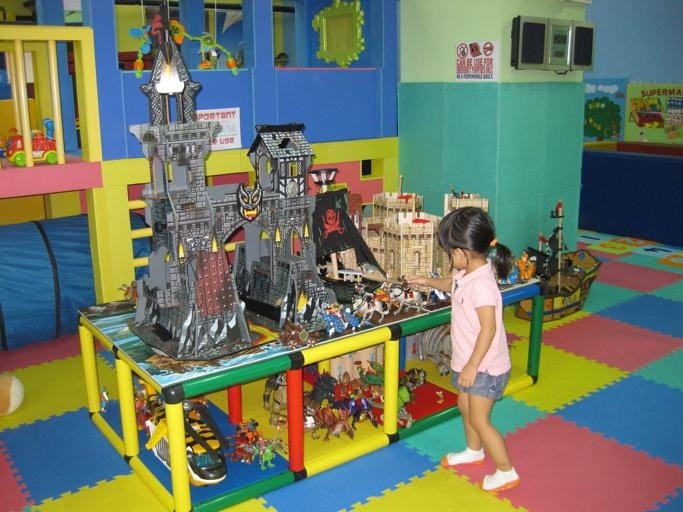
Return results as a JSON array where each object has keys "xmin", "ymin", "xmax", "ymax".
[
  {"xmin": 515, "ymin": 16, "xmax": 549, "ymax": 70},
  {"xmin": 571, "ymin": 19, "xmax": 596, "ymax": 68}
]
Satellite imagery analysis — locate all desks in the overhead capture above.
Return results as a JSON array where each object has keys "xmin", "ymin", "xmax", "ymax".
[{"xmin": 75, "ymin": 273, "xmax": 545, "ymax": 510}]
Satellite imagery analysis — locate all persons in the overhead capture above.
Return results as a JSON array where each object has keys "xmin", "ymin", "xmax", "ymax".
[
  {"xmin": 331, "ymin": 370, "xmax": 385, "ymax": 427},
  {"xmin": 280, "ymin": 272, "xmax": 449, "ymax": 348},
  {"xmin": 404, "ymin": 206, "xmax": 519, "ymax": 493},
  {"xmin": 226, "ymin": 418, "xmax": 276, "ymax": 471}
]
